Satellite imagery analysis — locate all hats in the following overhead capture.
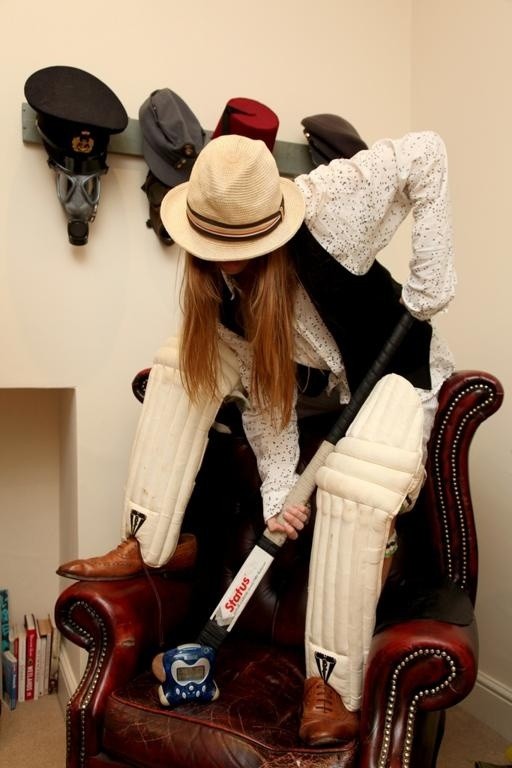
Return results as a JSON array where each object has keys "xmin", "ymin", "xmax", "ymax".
[
  {"xmin": 300, "ymin": 113, "xmax": 368, "ymax": 165},
  {"xmin": 138, "ymin": 86, "xmax": 204, "ymax": 187},
  {"xmin": 24, "ymin": 65, "xmax": 129, "ymax": 175},
  {"xmin": 210, "ymin": 98, "xmax": 280, "ymax": 154},
  {"xmin": 159, "ymin": 134, "xmax": 305, "ymax": 263}
]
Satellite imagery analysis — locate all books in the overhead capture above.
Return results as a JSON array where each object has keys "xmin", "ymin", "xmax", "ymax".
[{"xmin": 0, "ymin": 588, "xmax": 63, "ymax": 710}]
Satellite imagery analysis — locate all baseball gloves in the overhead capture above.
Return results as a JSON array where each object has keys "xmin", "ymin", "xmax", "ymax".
[{"xmin": 233, "ymin": 653, "xmax": 305, "ymax": 726}]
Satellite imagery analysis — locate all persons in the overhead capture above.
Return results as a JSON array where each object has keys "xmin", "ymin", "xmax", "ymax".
[{"xmin": 58, "ymin": 131, "xmax": 457, "ymax": 748}]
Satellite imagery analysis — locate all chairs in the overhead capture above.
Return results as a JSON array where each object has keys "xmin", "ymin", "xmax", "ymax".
[{"xmin": 52, "ymin": 365, "xmax": 505, "ymax": 767}]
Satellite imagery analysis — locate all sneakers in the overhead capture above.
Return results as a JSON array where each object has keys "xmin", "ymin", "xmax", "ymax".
[
  {"xmin": 56, "ymin": 533, "xmax": 197, "ymax": 583},
  {"xmin": 299, "ymin": 672, "xmax": 356, "ymax": 745}
]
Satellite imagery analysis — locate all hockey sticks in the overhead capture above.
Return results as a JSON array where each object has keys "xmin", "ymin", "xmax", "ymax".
[{"xmin": 152, "ymin": 313, "xmax": 413, "ymax": 682}]
